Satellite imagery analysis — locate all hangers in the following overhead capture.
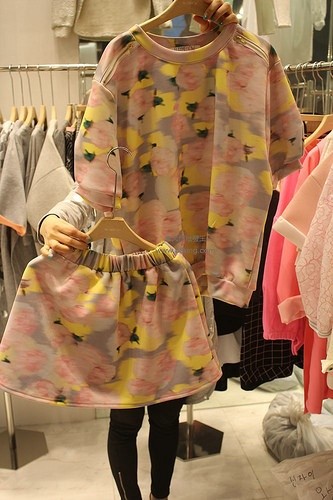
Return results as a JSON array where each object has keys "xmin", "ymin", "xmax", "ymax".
[
  {"xmin": 110, "ymin": 0, "xmax": 275, "ymax": 56},
  {"xmin": 283, "ymin": 59, "xmax": 333, "ymax": 160},
  {"xmin": 27, "ymin": 146, "xmax": 192, "ymax": 271},
  {"xmin": 0, "ymin": 65, "xmax": 93, "ymax": 138}
]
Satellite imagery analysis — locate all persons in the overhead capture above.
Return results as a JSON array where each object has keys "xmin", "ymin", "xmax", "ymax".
[{"xmin": 36, "ymin": 1, "xmax": 242, "ymax": 500}]
